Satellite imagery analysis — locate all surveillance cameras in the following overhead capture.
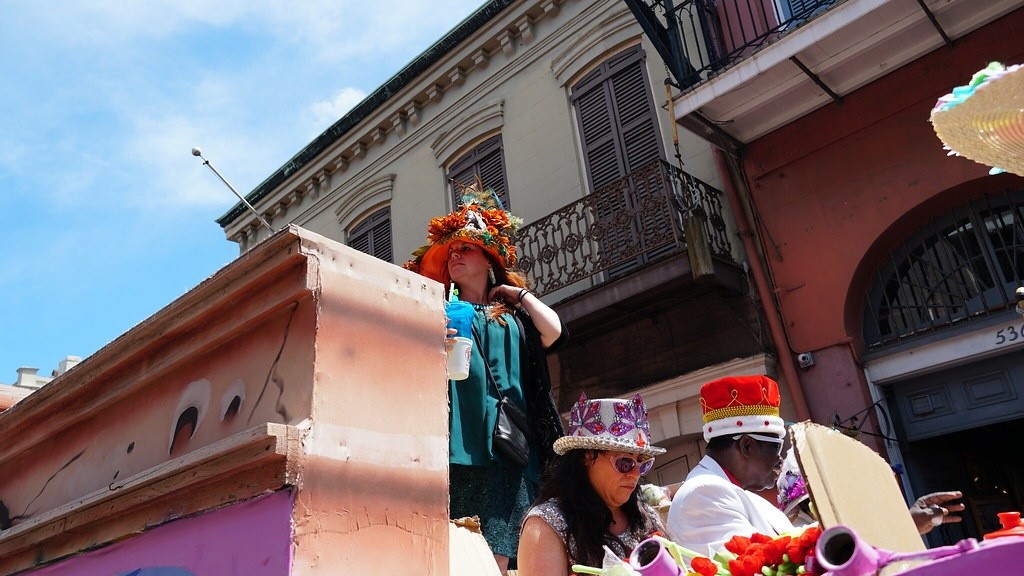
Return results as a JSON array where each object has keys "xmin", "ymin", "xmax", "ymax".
[{"xmin": 798, "ymin": 354, "xmax": 810, "ymax": 364}]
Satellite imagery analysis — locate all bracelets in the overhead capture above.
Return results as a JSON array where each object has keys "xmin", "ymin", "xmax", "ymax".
[{"xmin": 518, "ymin": 287, "xmax": 530, "ymax": 302}]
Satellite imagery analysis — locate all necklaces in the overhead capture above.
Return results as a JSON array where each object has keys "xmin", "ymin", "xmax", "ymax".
[{"xmin": 463, "ymin": 300, "xmax": 488, "ymax": 311}]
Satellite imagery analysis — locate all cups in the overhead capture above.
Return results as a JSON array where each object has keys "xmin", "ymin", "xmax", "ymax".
[
  {"xmin": 445, "ymin": 301, "xmax": 474, "ymax": 339},
  {"xmin": 446, "ymin": 337, "xmax": 472, "ymax": 380},
  {"xmin": 997, "ymin": 512, "xmax": 1020, "ymax": 529}
]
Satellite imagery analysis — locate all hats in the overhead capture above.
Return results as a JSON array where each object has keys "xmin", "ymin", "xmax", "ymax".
[
  {"xmin": 552, "ymin": 392, "xmax": 666, "ymax": 456},
  {"xmin": 400, "ymin": 174, "xmax": 525, "ymax": 286},
  {"xmin": 930, "ymin": 60, "xmax": 1024, "ymax": 177},
  {"xmin": 776, "ymin": 448, "xmax": 809, "ymax": 516},
  {"xmin": 699, "ymin": 376, "xmax": 787, "ymax": 443}
]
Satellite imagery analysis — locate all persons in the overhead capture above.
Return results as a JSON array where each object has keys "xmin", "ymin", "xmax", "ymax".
[
  {"xmin": 402, "ymin": 183, "xmax": 566, "ymax": 576},
  {"xmin": 776, "ymin": 448, "xmax": 817, "ymax": 528},
  {"xmin": 843, "ymin": 425, "xmax": 863, "ymax": 443},
  {"xmin": 666, "ymin": 375, "xmax": 965, "ymax": 561},
  {"xmin": 517, "ymin": 392, "xmax": 670, "ymax": 575}
]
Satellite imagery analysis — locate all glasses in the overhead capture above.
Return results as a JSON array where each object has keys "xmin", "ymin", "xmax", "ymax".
[
  {"xmin": 601, "ymin": 452, "xmax": 655, "ymax": 477},
  {"xmin": 732, "ymin": 433, "xmax": 785, "ymax": 457}
]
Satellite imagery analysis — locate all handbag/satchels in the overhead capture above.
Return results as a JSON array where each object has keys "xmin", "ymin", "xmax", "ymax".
[{"xmin": 492, "ymin": 397, "xmax": 532, "ymax": 468}]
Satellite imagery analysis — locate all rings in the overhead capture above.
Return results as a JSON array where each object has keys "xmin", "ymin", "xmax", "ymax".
[{"xmin": 931, "ymin": 505, "xmax": 943, "ymax": 515}]
